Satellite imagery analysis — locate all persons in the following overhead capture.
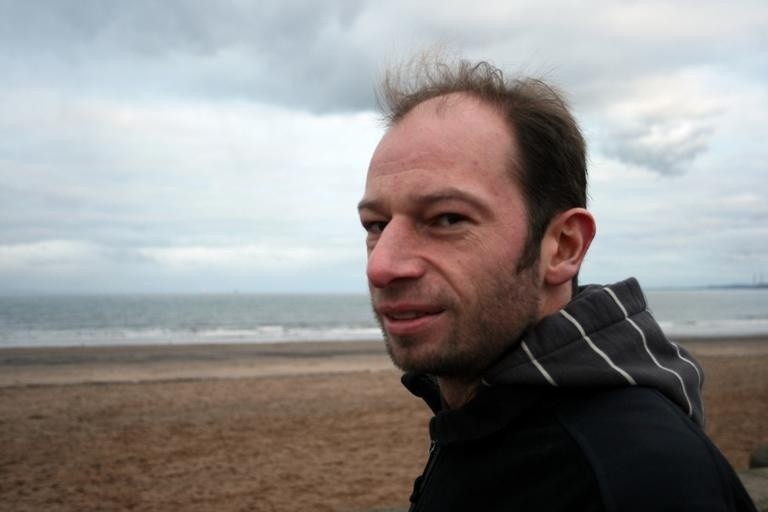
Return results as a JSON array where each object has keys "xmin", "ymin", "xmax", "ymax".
[{"xmin": 358, "ymin": 72, "xmax": 757, "ymax": 511}]
